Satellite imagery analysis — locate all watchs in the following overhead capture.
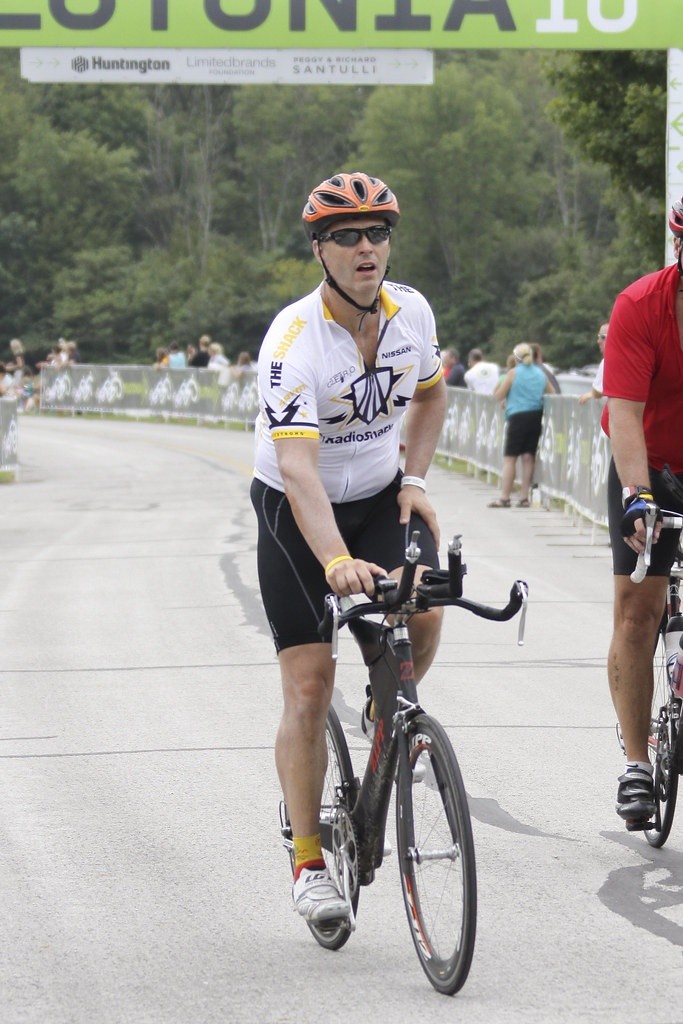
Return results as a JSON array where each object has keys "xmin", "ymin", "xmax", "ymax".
[{"xmin": 621, "ymin": 485, "xmax": 652, "ymax": 510}]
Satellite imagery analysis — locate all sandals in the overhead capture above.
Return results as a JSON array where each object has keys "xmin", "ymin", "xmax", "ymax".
[
  {"xmin": 516, "ymin": 499, "xmax": 530, "ymax": 508},
  {"xmin": 490, "ymin": 499, "xmax": 511, "ymax": 508}
]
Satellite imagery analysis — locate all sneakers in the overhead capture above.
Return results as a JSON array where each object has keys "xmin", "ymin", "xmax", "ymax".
[
  {"xmin": 361, "ymin": 683, "xmax": 426, "ymax": 784},
  {"xmin": 291, "ymin": 868, "xmax": 352, "ymax": 923},
  {"xmin": 617, "ymin": 769, "xmax": 657, "ymax": 821}
]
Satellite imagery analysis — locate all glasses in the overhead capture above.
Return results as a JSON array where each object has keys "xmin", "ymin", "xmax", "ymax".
[{"xmin": 317, "ymin": 223, "xmax": 391, "ymax": 249}]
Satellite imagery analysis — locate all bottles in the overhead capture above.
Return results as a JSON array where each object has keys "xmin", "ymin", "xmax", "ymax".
[
  {"xmin": 667, "ymin": 612, "xmax": 683, "ymax": 682},
  {"xmin": 669, "ymin": 635, "xmax": 682, "ymax": 698}
]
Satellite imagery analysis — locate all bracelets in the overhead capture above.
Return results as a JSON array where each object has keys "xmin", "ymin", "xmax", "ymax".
[
  {"xmin": 401, "ymin": 476, "xmax": 427, "ymax": 493},
  {"xmin": 324, "ymin": 555, "xmax": 353, "ymax": 576}
]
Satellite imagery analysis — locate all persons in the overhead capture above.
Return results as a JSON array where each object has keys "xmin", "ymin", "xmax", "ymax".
[
  {"xmin": 601, "ymin": 195, "xmax": 683, "ymax": 823},
  {"xmin": 247, "ymin": 173, "xmax": 450, "ymax": 925},
  {"xmin": 487, "ymin": 342, "xmax": 556, "ymax": 508},
  {"xmin": 441, "ymin": 321, "xmax": 609, "ymax": 405},
  {"xmin": 1, "ymin": 335, "xmax": 253, "ymax": 413}
]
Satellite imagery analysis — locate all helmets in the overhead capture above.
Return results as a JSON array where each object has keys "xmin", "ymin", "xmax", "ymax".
[
  {"xmin": 301, "ymin": 169, "xmax": 400, "ymax": 235},
  {"xmin": 670, "ymin": 195, "xmax": 683, "ymax": 237}
]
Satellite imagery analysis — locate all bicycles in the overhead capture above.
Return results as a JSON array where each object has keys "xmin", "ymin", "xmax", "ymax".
[
  {"xmin": 616, "ymin": 500, "xmax": 683, "ymax": 848},
  {"xmin": 280, "ymin": 533, "xmax": 530, "ymax": 996}
]
